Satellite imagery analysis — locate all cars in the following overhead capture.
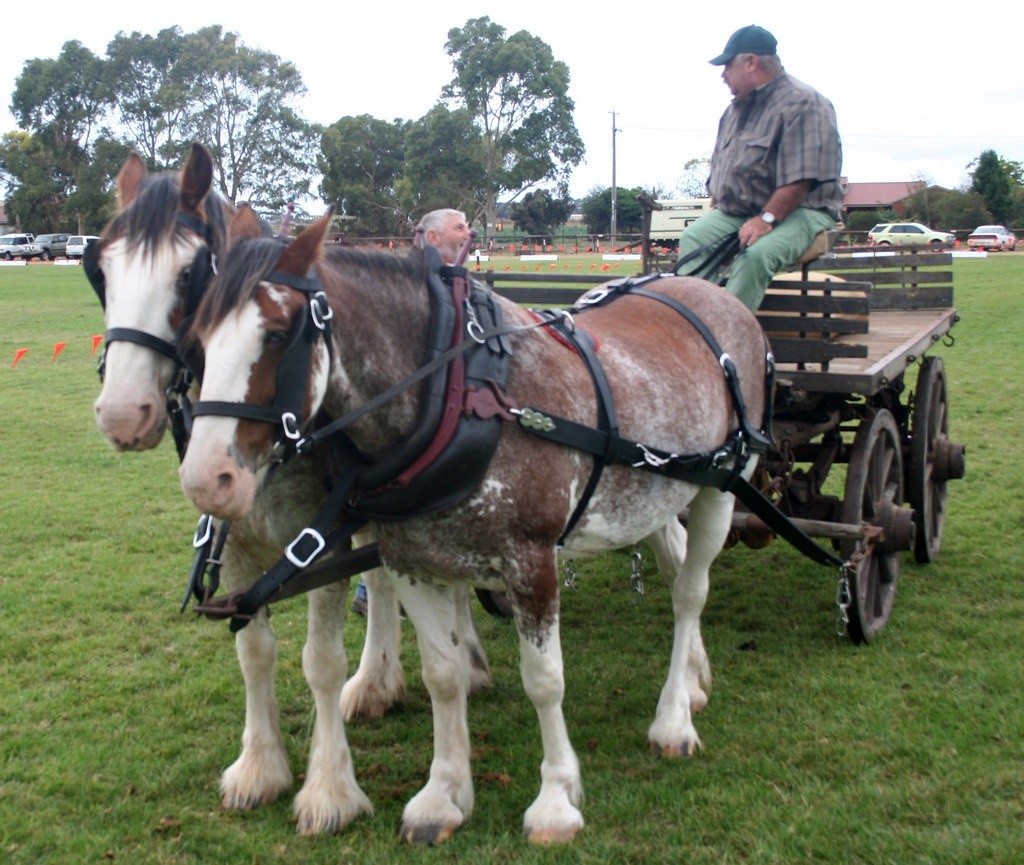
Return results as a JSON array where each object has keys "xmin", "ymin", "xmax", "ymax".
[{"xmin": 967, "ymin": 225, "xmax": 1016, "ymax": 251}]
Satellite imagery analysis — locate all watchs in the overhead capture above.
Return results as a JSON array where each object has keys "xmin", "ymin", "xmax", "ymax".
[{"xmin": 759, "ymin": 211, "xmax": 780, "ymax": 230}]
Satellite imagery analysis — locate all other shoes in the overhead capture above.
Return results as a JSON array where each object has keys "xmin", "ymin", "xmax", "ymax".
[{"xmin": 351, "ymin": 584, "xmax": 371, "ymax": 616}]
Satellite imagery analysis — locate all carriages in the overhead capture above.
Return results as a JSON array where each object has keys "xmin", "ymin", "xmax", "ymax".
[{"xmin": 82, "ymin": 141, "xmax": 967, "ymax": 847}]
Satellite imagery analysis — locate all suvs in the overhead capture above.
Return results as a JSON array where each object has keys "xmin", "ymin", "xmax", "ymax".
[
  {"xmin": 0, "ymin": 233, "xmax": 34, "ymax": 260},
  {"xmin": 65, "ymin": 235, "xmax": 101, "ymax": 259},
  {"xmin": 19, "ymin": 233, "xmax": 71, "ymax": 262},
  {"xmin": 867, "ymin": 222, "xmax": 956, "ymax": 251}
]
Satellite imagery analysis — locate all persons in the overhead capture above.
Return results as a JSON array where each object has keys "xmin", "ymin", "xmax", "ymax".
[
  {"xmin": 350, "ymin": 207, "xmax": 471, "ymax": 618},
  {"xmin": 678, "ymin": 24, "xmax": 843, "ymax": 315}
]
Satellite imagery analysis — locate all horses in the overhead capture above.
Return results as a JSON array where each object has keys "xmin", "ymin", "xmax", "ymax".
[{"xmin": 93, "ymin": 137, "xmax": 774, "ymax": 849}]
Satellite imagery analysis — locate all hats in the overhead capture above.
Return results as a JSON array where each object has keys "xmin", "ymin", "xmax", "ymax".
[{"xmin": 707, "ymin": 25, "xmax": 777, "ymax": 65}]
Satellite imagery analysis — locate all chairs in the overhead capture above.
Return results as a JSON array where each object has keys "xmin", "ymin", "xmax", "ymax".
[{"xmin": 782, "ymin": 222, "xmax": 847, "ymax": 274}]
